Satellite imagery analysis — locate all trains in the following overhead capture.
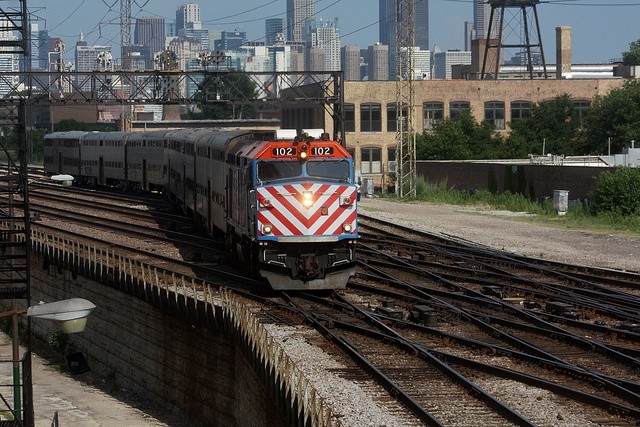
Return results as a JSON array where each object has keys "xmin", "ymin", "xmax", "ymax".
[{"xmin": 43, "ymin": 127, "xmax": 361, "ymax": 290}]
